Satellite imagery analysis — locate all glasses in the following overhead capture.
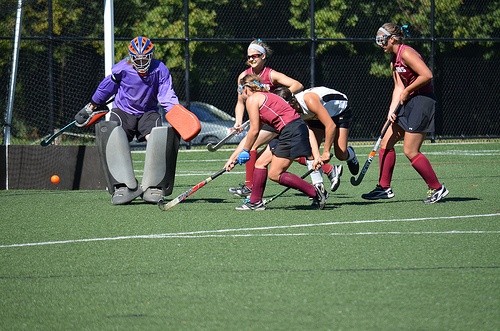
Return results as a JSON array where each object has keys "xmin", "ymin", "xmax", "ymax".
[
  {"xmin": 236, "ymin": 84, "xmax": 248, "ymax": 95},
  {"xmin": 376, "ymin": 35, "xmax": 392, "ymax": 46},
  {"xmin": 246, "ymin": 53, "xmax": 263, "ymax": 59}
]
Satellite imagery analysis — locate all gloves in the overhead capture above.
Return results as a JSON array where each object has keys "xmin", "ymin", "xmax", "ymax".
[{"xmin": 237, "ymin": 148, "xmax": 250, "ymax": 165}]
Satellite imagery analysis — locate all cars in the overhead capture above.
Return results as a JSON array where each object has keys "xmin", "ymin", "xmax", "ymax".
[{"xmin": 127, "ymin": 101, "xmax": 244, "ymax": 145}]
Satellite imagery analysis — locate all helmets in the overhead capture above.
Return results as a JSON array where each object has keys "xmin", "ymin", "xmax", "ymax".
[{"xmin": 128, "ymin": 37, "xmax": 155, "ymax": 59}]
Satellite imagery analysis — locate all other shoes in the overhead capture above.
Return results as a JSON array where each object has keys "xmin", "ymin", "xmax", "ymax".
[
  {"xmin": 112, "ymin": 178, "xmax": 143, "ymax": 205},
  {"xmin": 140, "ymin": 186, "xmax": 164, "ymax": 203}
]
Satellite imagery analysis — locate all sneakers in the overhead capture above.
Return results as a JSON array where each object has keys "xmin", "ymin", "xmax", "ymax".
[
  {"xmin": 234, "ymin": 200, "xmax": 266, "ymax": 211},
  {"xmin": 346, "ymin": 146, "xmax": 360, "ymax": 176},
  {"xmin": 309, "ymin": 183, "xmax": 328, "ymax": 209},
  {"xmin": 229, "ymin": 182, "xmax": 252, "ymax": 198},
  {"xmin": 361, "ymin": 184, "xmax": 394, "ymax": 200},
  {"xmin": 424, "ymin": 184, "xmax": 449, "ymax": 204},
  {"xmin": 326, "ymin": 164, "xmax": 343, "ymax": 192}
]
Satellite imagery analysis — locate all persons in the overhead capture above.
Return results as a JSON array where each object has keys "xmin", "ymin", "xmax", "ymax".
[
  {"xmin": 74, "ymin": 36, "xmax": 201, "ymax": 207},
  {"xmin": 223, "ymin": 73, "xmax": 326, "ymax": 211},
  {"xmin": 273, "ymin": 86, "xmax": 360, "ymax": 199},
  {"xmin": 360, "ymin": 22, "xmax": 449, "ymax": 203},
  {"xmin": 233, "ymin": 39, "xmax": 304, "ymax": 199}
]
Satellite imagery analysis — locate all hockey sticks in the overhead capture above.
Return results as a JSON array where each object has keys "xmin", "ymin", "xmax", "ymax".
[
  {"xmin": 263, "ymin": 153, "xmax": 334, "ymax": 205},
  {"xmin": 39, "ymin": 96, "xmax": 115, "ymax": 148},
  {"xmin": 350, "ymin": 100, "xmax": 401, "ymax": 187},
  {"xmin": 207, "ymin": 119, "xmax": 251, "ymax": 152},
  {"xmin": 158, "ymin": 161, "xmax": 239, "ymax": 211}
]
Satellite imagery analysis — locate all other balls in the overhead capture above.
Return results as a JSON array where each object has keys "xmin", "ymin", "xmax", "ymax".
[{"xmin": 51, "ymin": 175, "xmax": 60, "ymax": 184}]
